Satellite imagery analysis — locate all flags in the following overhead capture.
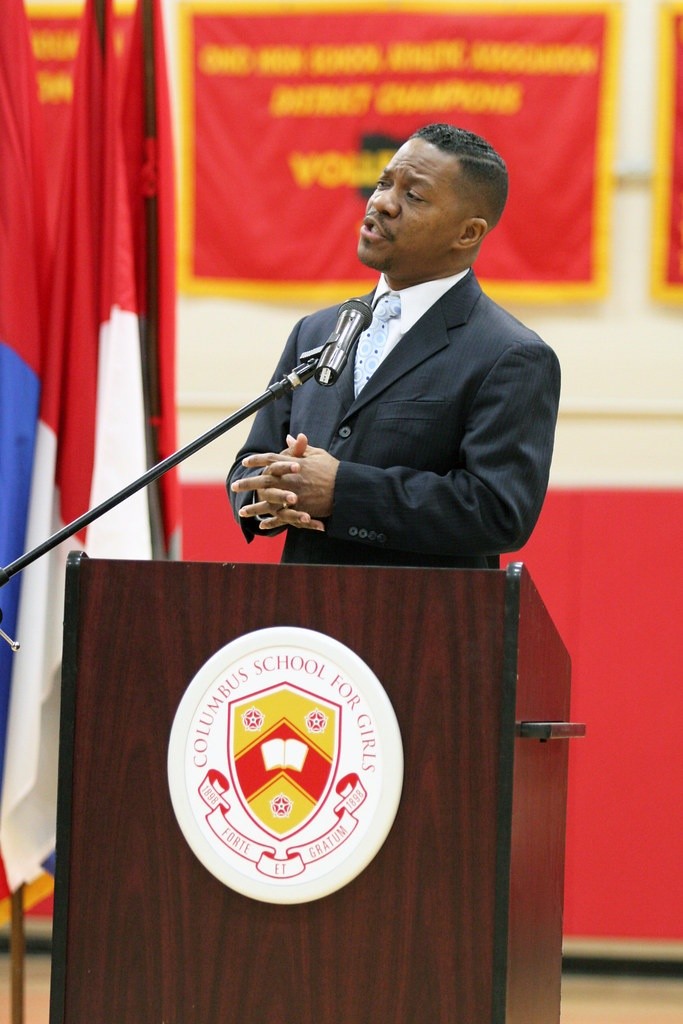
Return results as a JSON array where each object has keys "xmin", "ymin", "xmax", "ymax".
[{"xmin": 0, "ymin": 0, "xmax": 184, "ymax": 928}]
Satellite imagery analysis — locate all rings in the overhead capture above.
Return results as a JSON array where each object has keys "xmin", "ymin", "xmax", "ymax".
[{"xmin": 283, "ymin": 503, "xmax": 288, "ymax": 508}]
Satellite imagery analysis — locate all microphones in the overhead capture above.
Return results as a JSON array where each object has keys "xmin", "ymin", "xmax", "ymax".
[{"xmin": 314, "ymin": 298, "xmax": 373, "ymax": 387}]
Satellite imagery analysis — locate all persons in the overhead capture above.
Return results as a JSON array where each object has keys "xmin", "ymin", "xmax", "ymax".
[{"xmin": 226, "ymin": 123, "xmax": 562, "ymax": 569}]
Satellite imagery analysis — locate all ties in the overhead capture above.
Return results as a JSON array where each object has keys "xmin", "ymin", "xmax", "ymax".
[{"xmin": 350, "ymin": 293, "xmax": 403, "ymax": 402}]
